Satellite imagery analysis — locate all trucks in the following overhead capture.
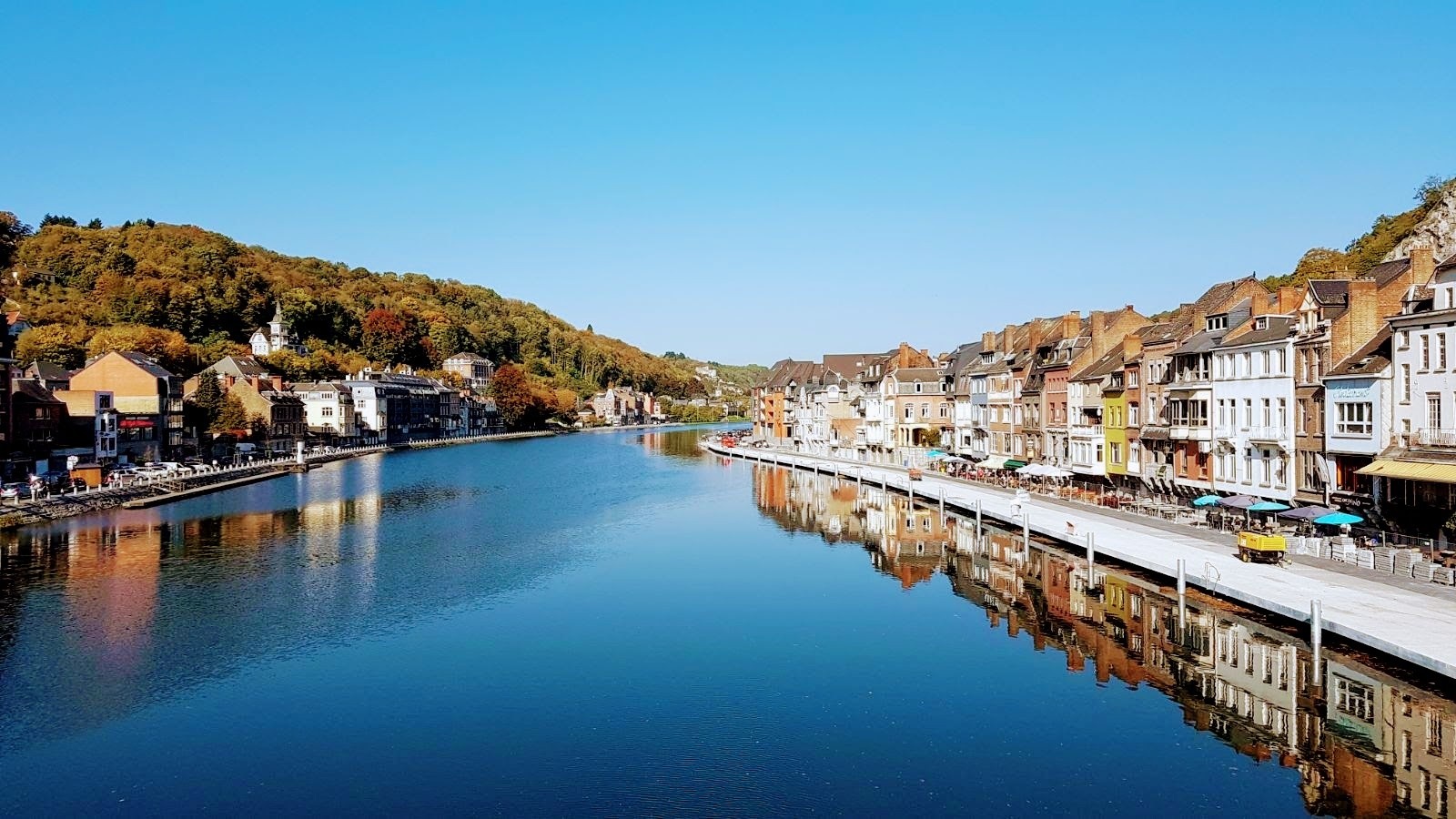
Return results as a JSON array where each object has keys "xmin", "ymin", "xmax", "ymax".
[
  {"xmin": 909, "ymin": 468, "xmax": 923, "ymax": 480},
  {"xmin": 1237, "ymin": 529, "xmax": 1292, "ymax": 565}
]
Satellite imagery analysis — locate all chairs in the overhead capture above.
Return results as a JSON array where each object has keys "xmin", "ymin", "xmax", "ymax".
[{"xmin": 1207, "ymin": 515, "xmax": 1280, "ymax": 535}]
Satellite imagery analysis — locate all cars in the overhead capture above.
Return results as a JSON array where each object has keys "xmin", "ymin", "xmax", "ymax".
[
  {"xmin": 0, "ymin": 470, "xmax": 87, "ymax": 501},
  {"xmin": 303, "ymin": 445, "xmax": 335, "ymax": 452},
  {"xmin": 103, "ymin": 461, "xmax": 214, "ymax": 487},
  {"xmin": 213, "ymin": 455, "xmax": 248, "ymax": 468}
]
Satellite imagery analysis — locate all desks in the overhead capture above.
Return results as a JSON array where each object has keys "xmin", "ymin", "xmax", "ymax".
[
  {"xmin": 1214, "ymin": 519, "xmax": 1225, "ymax": 522},
  {"xmin": 952, "ymin": 470, "xmax": 1195, "ymax": 522}
]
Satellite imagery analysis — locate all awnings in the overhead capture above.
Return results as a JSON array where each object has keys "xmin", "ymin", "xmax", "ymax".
[
  {"xmin": 1354, "ymin": 459, "xmax": 1456, "ymax": 485},
  {"xmin": 1004, "ymin": 461, "xmax": 1025, "ymax": 469},
  {"xmin": 50, "ymin": 447, "xmax": 93, "ymax": 457}
]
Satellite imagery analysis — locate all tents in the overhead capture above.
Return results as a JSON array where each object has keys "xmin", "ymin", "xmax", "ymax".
[
  {"xmin": 975, "ymin": 459, "xmax": 1006, "ymax": 486},
  {"xmin": 1015, "ymin": 463, "xmax": 1075, "ymax": 497}
]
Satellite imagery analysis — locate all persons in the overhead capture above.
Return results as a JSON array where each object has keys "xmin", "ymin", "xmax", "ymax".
[
  {"xmin": 949, "ymin": 462, "xmax": 954, "ymax": 471},
  {"xmin": 931, "ymin": 462, "xmax": 934, "ymax": 468},
  {"xmin": 998, "ymin": 474, "xmax": 1006, "ymax": 486},
  {"xmin": 1206, "ymin": 511, "xmax": 1262, "ymax": 532}
]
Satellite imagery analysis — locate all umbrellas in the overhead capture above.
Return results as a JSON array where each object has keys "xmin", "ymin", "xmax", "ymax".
[
  {"xmin": 1248, "ymin": 502, "xmax": 1290, "ymax": 522},
  {"xmin": 1313, "ymin": 512, "xmax": 1364, "ymax": 535},
  {"xmin": 1193, "ymin": 495, "xmax": 1223, "ymax": 512},
  {"xmin": 1277, "ymin": 505, "xmax": 1335, "ymax": 537},
  {"xmin": 925, "ymin": 451, "xmax": 974, "ymax": 479},
  {"xmin": 1219, "ymin": 494, "xmax": 1265, "ymax": 517}
]
